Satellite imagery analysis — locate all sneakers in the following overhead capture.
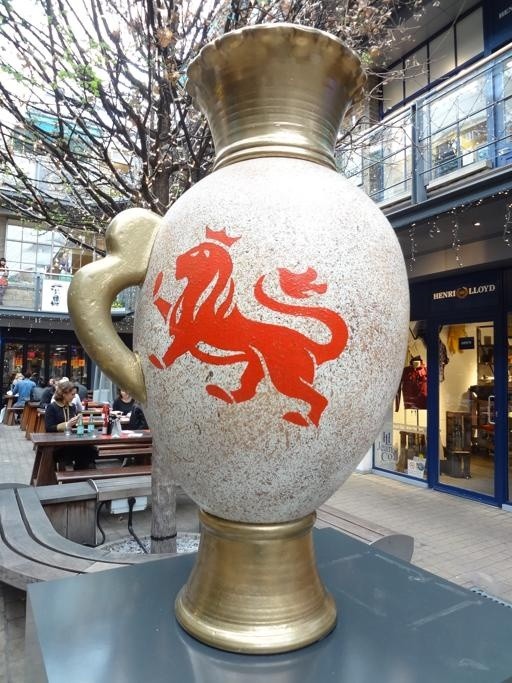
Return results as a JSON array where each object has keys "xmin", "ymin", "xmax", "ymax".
[{"xmin": 121, "ymin": 458, "xmax": 129, "ymax": 467}]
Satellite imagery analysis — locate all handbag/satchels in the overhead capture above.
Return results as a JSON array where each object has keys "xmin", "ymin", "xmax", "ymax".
[
  {"xmin": 111, "ymin": 413, "xmax": 121, "ymax": 435},
  {"xmin": 31, "ymin": 377, "xmax": 52, "ymax": 402}
]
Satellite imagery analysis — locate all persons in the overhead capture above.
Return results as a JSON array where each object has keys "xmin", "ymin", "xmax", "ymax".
[
  {"xmin": 5, "ymin": 371, "xmax": 148, "ymax": 471},
  {"xmin": 0, "ymin": 257, "xmax": 9, "ymax": 305}
]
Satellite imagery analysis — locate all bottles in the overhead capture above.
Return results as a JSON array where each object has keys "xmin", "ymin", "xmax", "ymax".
[
  {"xmin": 103, "ymin": 401, "xmax": 109, "ymax": 415},
  {"xmin": 76, "ymin": 409, "xmax": 124, "ymax": 437},
  {"xmin": 82, "ymin": 399, "xmax": 88, "ymax": 410}
]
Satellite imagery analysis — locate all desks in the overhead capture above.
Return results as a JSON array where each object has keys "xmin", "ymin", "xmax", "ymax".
[{"xmin": 3, "ymin": 395, "xmax": 152, "ymax": 486}]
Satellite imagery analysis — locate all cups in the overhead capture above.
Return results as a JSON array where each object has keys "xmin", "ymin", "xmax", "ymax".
[{"xmin": 64, "ymin": 420, "xmax": 72, "ymax": 436}]
